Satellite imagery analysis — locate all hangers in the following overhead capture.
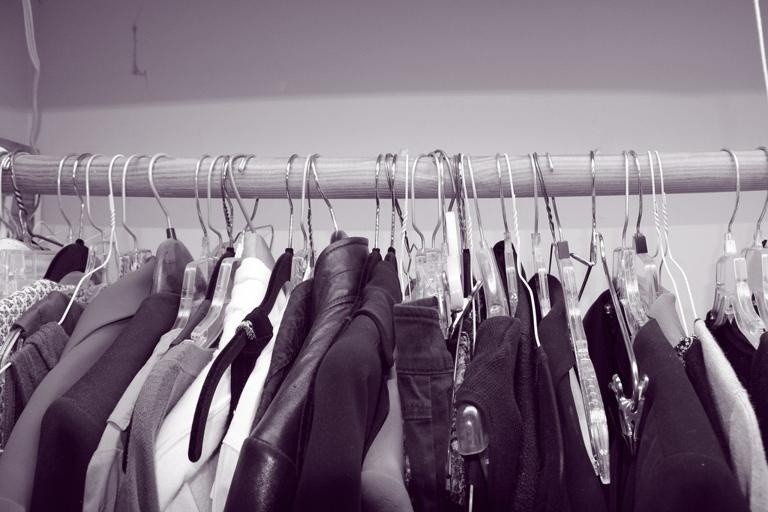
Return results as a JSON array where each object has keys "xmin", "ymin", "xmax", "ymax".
[{"xmin": 0, "ymin": 147, "xmax": 768, "ymax": 486}]
